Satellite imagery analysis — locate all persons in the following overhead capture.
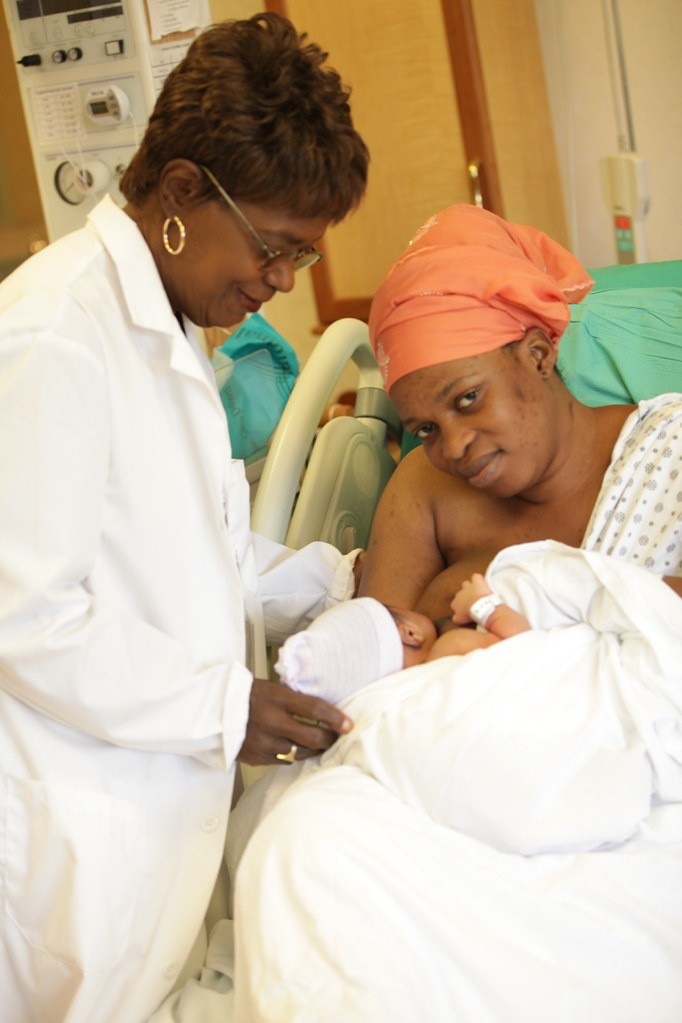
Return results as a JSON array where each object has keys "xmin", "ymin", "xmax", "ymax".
[
  {"xmin": 0, "ymin": 10, "xmax": 364, "ymax": 1023},
  {"xmin": 274, "ymin": 572, "xmax": 532, "ymax": 709},
  {"xmin": 359, "ymin": 206, "xmax": 682, "ymax": 604}
]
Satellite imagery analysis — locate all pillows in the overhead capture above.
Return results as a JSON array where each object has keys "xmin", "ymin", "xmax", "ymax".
[{"xmin": 556, "ymin": 286, "xmax": 682, "ymax": 408}]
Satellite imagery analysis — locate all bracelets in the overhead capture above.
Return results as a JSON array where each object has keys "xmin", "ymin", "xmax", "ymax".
[{"xmin": 470, "ymin": 593, "xmax": 503, "ymax": 627}]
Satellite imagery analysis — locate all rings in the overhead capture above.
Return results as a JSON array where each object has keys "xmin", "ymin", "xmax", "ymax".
[{"xmin": 276, "ymin": 744, "xmax": 298, "ymax": 763}]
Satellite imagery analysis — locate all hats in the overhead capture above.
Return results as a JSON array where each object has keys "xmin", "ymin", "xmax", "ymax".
[{"xmin": 274, "ymin": 597, "xmax": 404, "ymax": 708}]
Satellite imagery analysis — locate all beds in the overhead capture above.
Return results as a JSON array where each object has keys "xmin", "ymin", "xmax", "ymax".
[{"xmin": 237, "ymin": 258, "xmax": 682, "ymax": 1022}]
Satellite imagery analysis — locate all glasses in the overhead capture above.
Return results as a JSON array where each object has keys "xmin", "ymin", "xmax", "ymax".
[{"xmin": 203, "ymin": 166, "xmax": 324, "ymax": 272}]
